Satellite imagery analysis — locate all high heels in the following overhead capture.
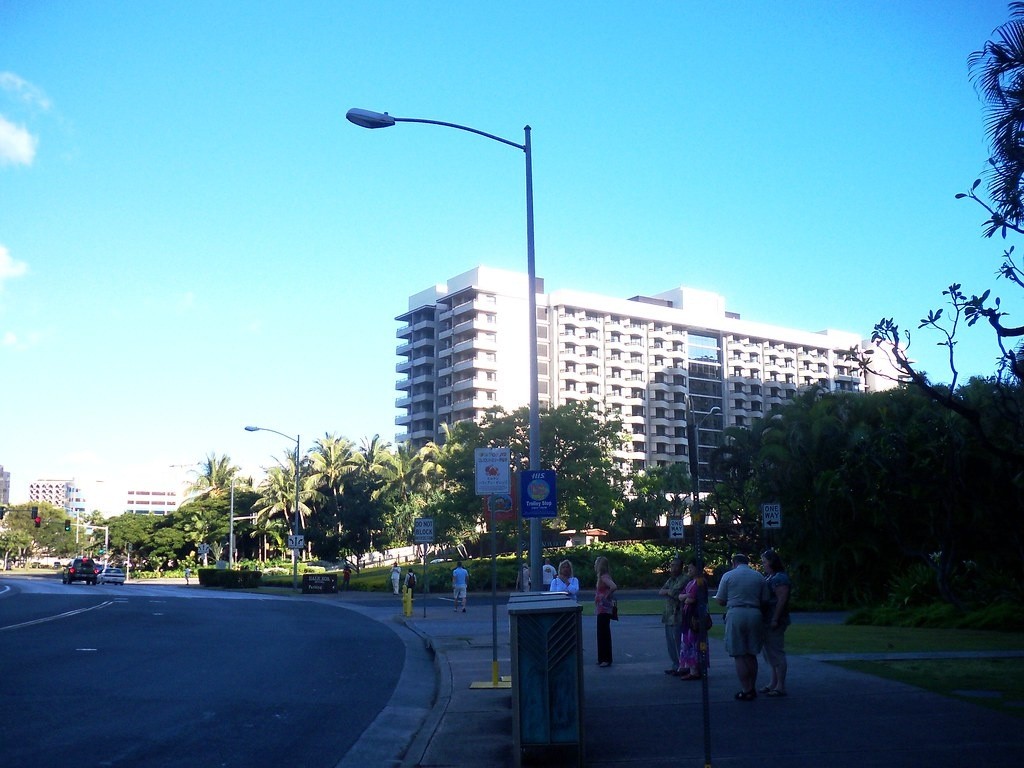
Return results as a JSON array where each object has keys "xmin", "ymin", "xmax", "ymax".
[
  {"xmin": 596, "ymin": 662, "xmax": 602, "ymax": 665},
  {"xmin": 599, "ymin": 661, "xmax": 611, "ymax": 667}
]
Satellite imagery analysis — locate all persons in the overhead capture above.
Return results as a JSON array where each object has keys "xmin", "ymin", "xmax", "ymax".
[
  {"xmin": 340, "ymin": 566, "xmax": 351, "ymax": 592},
  {"xmin": 672, "ymin": 558, "xmax": 711, "ymax": 681},
  {"xmin": 657, "ymin": 559, "xmax": 690, "ymax": 676},
  {"xmin": 550, "ymin": 559, "xmax": 579, "ymax": 602},
  {"xmin": 759, "ymin": 550, "xmax": 792, "ymax": 697},
  {"xmin": 516, "ymin": 562, "xmax": 531, "ymax": 592},
  {"xmin": 542, "ymin": 558, "xmax": 557, "ymax": 592},
  {"xmin": 390, "ymin": 562, "xmax": 401, "ymax": 596},
  {"xmin": 593, "ymin": 556, "xmax": 617, "ymax": 667},
  {"xmin": 716, "ymin": 552, "xmax": 771, "ymax": 701},
  {"xmin": 404, "ymin": 568, "xmax": 417, "ymax": 604},
  {"xmin": 183, "ymin": 566, "xmax": 193, "ymax": 586},
  {"xmin": 451, "ymin": 562, "xmax": 469, "ymax": 612}
]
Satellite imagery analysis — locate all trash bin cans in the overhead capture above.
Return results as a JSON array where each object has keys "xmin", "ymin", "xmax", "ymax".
[
  {"xmin": 506, "ymin": 598, "xmax": 584, "ymax": 749},
  {"xmin": 509, "ymin": 591, "xmax": 572, "ymax": 597},
  {"xmin": 509, "ymin": 594, "xmax": 573, "ymax": 604}
]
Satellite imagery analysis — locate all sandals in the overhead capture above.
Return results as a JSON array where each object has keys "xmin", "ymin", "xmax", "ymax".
[
  {"xmin": 747, "ymin": 689, "xmax": 758, "ymax": 698},
  {"xmin": 673, "ymin": 669, "xmax": 689, "ymax": 675},
  {"xmin": 735, "ymin": 691, "xmax": 751, "ymax": 700},
  {"xmin": 681, "ymin": 674, "xmax": 700, "ymax": 680}
]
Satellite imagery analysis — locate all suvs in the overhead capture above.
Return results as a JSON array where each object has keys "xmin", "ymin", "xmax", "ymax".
[{"xmin": 61, "ymin": 558, "xmax": 98, "ymax": 585}]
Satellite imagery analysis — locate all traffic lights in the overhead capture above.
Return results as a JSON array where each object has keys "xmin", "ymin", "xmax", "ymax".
[
  {"xmin": 64, "ymin": 519, "xmax": 71, "ymax": 532},
  {"xmin": 34, "ymin": 516, "xmax": 41, "ymax": 528}
]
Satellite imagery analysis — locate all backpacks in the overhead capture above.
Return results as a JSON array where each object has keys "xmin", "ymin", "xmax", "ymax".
[{"xmin": 408, "ymin": 572, "xmax": 415, "ymax": 586}]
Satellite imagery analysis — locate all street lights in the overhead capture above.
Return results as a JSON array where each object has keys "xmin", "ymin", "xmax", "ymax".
[
  {"xmin": 344, "ymin": 105, "xmax": 544, "ymax": 592},
  {"xmin": 244, "ymin": 423, "xmax": 302, "ymax": 590},
  {"xmin": 689, "ymin": 405, "xmax": 722, "ymax": 544}
]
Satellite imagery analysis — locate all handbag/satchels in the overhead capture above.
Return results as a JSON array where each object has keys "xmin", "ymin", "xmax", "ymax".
[
  {"xmin": 691, "ymin": 613, "xmax": 713, "ymax": 632},
  {"xmin": 610, "ymin": 606, "xmax": 618, "ymax": 621}
]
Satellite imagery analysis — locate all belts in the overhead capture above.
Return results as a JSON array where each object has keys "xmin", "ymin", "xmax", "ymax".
[{"xmin": 728, "ymin": 604, "xmax": 758, "ymax": 609}]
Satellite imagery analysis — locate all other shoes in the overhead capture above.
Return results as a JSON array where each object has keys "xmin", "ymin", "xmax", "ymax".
[{"xmin": 462, "ymin": 608, "xmax": 467, "ymax": 612}]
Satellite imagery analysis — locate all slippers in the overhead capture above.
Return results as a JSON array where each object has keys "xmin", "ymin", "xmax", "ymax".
[
  {"xmin": 766, "ymin": 690, "xmax": 788, "ymax": 697},
  {"xmin": 758, "ymin": 686, "xmax": 772, "ymax": 693}
]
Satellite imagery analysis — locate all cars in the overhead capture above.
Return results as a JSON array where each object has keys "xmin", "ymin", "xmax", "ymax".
[{"xmin": 96, "ymin": 568, "xmax": 126, "ymax": 586}]
